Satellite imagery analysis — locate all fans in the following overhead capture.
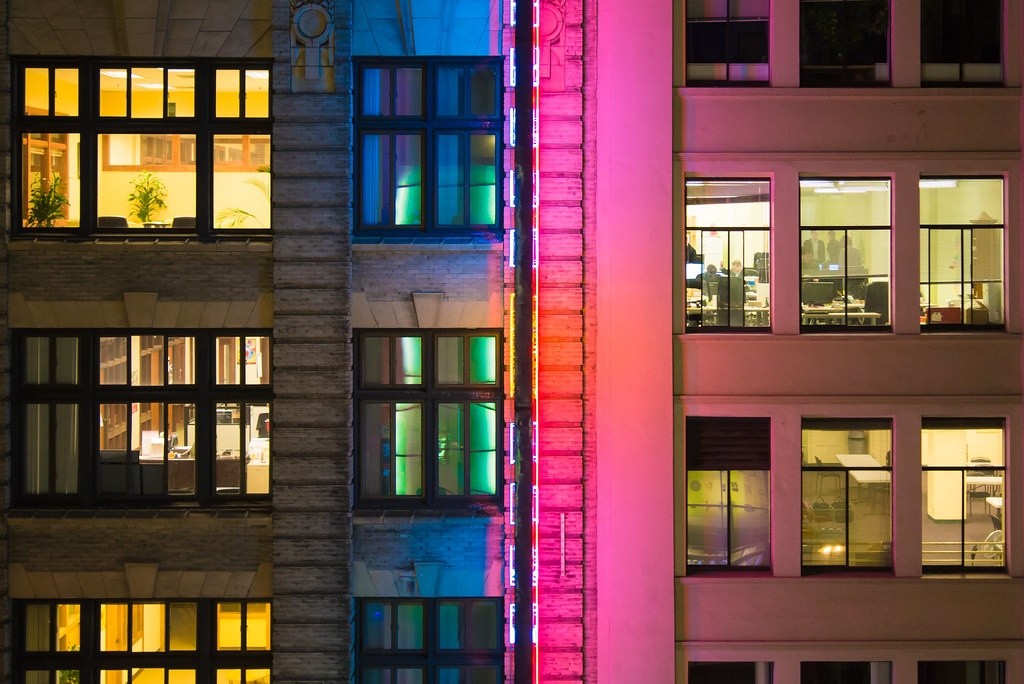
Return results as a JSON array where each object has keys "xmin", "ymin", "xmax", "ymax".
[{"xmin": 982, "ymin": 529, "xmax": 1002, "ymax": 558}]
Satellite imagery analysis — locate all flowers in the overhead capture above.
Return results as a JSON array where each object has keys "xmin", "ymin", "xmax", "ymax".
[{"xmin": 126, "ymin": 169, "xmax": 168, "ymax": 222}]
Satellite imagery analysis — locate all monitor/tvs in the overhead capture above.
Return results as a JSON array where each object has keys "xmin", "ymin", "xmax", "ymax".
[
  {"xmin": 802, "ymin": 282, "xmax": 833, "ymax": 306},
  {"xmin": 715, "ymin": 272, "xmax": 723, "ymax": 278}
]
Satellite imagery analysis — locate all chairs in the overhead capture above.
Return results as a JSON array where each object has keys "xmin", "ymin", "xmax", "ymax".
[
  {"xmin": 859, "ymin": 281, "xmax": 888, "ymax": 324},
  {"xmin": 716, "ymin": 277, "xmax": 746, "ymax": 326},
  {"xmin": 990, "ymin": 514, "xmax": 1001, "ymax": 530},
  {"xmin": 970, "ymin": 457, "xmax": 995, "ymax": 496}
]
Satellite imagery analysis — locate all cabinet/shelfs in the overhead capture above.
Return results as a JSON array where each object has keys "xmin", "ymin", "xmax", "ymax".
[
  {"xmin": 100, "ymin": 338, "xmax": 195, "ymax": 450},
  {"xmin": 22, "ymin": 107, "xmax": 70, "ymax": 221}
]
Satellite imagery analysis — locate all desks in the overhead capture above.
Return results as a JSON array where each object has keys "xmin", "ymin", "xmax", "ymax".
[
  {"xmin": 966, "ymin": 476, "xmax": 1002, "ymax": 520},
  {"xmin": 139, "ymin": 449, "xmax": 250, "ymax": 493},
  {"xmin": 815, "ymin": 455, "xmax": 842, "ymax": 497},
  {"xmin": 836, "ymin": 453, "xmax": 891, "ymax": 518},
  {"xmin": 686, "ymin": 282, "xmax": 881, "ymax": 325}
]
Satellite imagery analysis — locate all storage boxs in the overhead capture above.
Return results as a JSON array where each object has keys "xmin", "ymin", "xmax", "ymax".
[
  {"xmin": 964, "ymin": 300, "xmax": 988, "ymax": 326},
  {"xmin": 812, "ymin": 500, "xmax": 854, "ymax": 521},
  {"xmin": 926, "ymin": 306, "xmax": 960, "ymax": 324}
]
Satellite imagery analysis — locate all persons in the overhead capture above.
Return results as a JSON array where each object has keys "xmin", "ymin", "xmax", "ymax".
[
  {"xmin": 801, "ymin": 230, "xmax": 862, "ymax": 267},
  {"xmin": 696, "ymin": 257, "xmax": 748, "ymax": 283}
]
